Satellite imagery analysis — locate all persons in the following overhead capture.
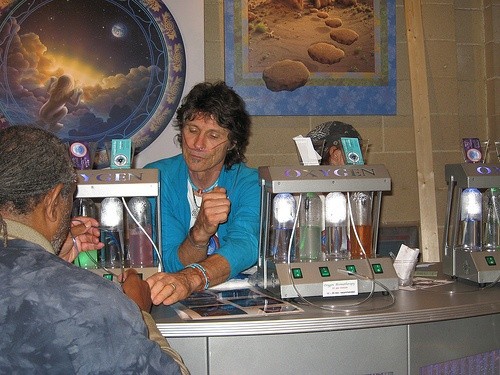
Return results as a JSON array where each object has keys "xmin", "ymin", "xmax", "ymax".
[
  {"xmin": 144, "ymin": 82, "xmax": 261, "ymax": 305},
  {"xmin": 0, "ymin": 126, "xmax": 191, "ymax": 375},
  {"xmin": 306, "ymin": 122, "xmax": 365, "ymax": 166}
]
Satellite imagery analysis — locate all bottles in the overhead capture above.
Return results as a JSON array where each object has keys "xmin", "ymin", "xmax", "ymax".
[
  {"xmin": 99, "ymin": 197, "xmax": 124, "ymax": 268},
  {"xmin": 72, "ymin": 198, "xmax": 98, "ymax": 269},
  {"xmin": 273, "ymin": 192, "xmax": 296, "ymax": 263},
  {"xmin": 126, "ymin": 197, "xmax": 154, "ymax": 267},
  {"xmin": 350, "ymin": 192, "xmax": 372, "ymax": 259},
  {"xmin": 300, "ymin": 193, "xmax": 322, "ymax": 261},
  {"xmin": 461, "ymin": 188, "xmax": 482, "ymax": 250},
  {"xmin": 325, "ymin": 192, "xmax": 348, "ymax": 260},
  {"xmin": 483, "ymin": 188, "xmax": 500, "ymax": 251}
]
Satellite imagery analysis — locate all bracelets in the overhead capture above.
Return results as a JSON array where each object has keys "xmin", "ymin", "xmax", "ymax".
[{"xmin": 185, "ymin": 263, "xmax": 209, "ymax": 296}]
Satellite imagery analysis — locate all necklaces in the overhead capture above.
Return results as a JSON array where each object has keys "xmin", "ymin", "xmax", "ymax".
[{"xmin": 187, "ymin": 177, "xmax": 222, "ymax": 195}]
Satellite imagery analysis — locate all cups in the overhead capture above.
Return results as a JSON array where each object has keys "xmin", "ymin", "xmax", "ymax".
[{"xmin": 395, "ymin": 260, "xmax": 417, "ymax": 287}]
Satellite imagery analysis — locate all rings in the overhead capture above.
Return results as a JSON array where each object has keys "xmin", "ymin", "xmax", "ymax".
[{"xmin": 169, "ymin": 282, "xmax": 179, "ymax": 296}]
